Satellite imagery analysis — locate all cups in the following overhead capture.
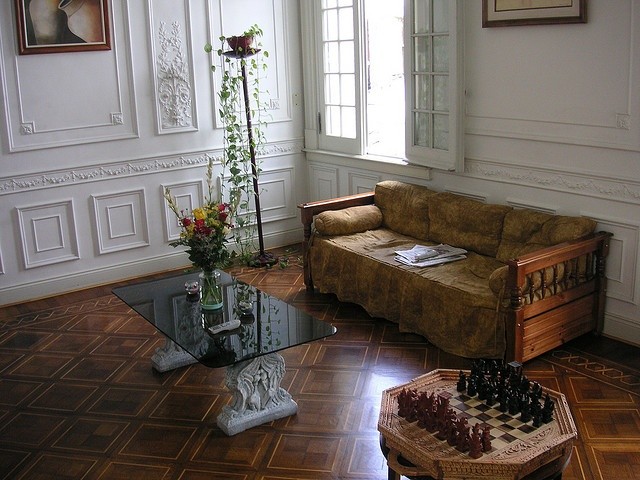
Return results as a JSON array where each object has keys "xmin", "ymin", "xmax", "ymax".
[
  {"xmin": 240, "ymin": 314, "xmax": 255, "ymax": 325},
  {"xmin": 186, "ymin": 295, "xmax": 200, "ymax": 303},
  {"xmin": 239, "ymin": 300, "xmax": 254, "ymax": 315},
  {"xmin": 185, "ymin": 280, "xmax": 202, "ymax": 294}
]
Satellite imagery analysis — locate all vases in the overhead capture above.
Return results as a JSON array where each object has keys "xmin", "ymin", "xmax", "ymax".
[{"xmin": 199, "ymin": 269, "xmax": 223, "ymax": 310}]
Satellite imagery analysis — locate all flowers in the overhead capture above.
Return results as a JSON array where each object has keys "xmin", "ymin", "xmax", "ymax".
[{"xmin": 163, "ymin": 155, "xmax": 245, "ymax": 304}]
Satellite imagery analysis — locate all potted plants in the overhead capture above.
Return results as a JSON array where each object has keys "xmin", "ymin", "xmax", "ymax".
[{"xmin": 204, "ymin": 24, "xmax": 291, "ymax": 270}]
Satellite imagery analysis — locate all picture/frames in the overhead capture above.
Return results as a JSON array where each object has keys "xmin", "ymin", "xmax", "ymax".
[
  {"xmin": 15, "ymin": 0, "xmax": 111, "ymax": 55},
  {"xmin": 482, "ymin": 0, "xmax": 587, "ymax": 28}
]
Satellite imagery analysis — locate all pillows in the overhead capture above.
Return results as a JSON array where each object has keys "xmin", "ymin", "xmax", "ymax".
[
  {"xmin": 314, "ymin": 205, "xmax": 383, "ymax": 235},
  {"xmin": 489, "ymin": 259, "xmax": 573, "ymax": 299}
]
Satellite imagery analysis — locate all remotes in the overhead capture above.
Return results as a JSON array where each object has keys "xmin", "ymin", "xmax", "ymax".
[
  {"xmin": 208, "ymin": 320, "xmax": 241, "ymax": 334},
  {"xmin": 415, "ymin": 250, "xmax": 438, "ymax": 260}
]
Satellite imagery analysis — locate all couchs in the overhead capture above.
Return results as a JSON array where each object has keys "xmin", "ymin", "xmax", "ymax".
[{"xmin": 297, "ymin": 181, "xmax": 614, "ymax": 365}]
